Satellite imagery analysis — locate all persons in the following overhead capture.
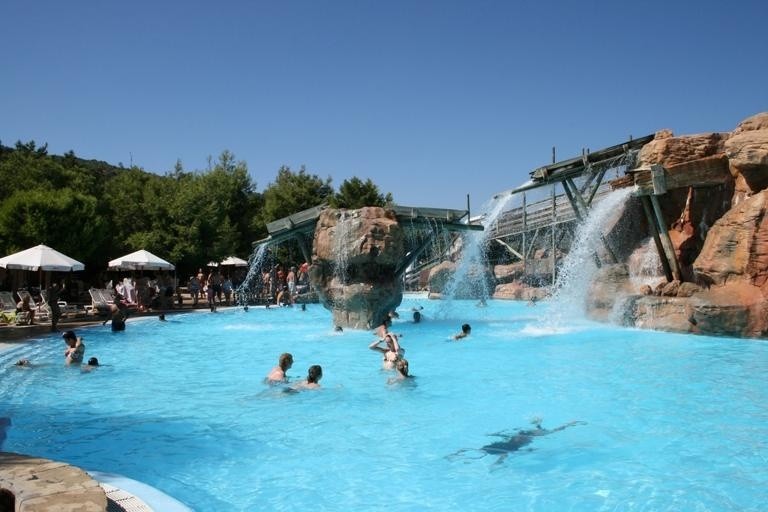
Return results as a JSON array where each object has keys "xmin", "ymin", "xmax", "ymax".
[
  {"xmin": 386, "ymin": 359, "xmax": 413, "ymax": 386},
  {"xmin": 286, "ymin": 265, "xmax": 296, "ymax": 296},
  {"xmin": 13, "ymin": 293, "xmax": 37, "ymax": 325},
  {"xmin": 243, "ymin": 305, "xmax": 247, "ymax": 312},
  {"xmin": 268, "ymin": 365, "xmax": 323, "ymax": 399},
  {"xmin": 203, "ymin": 285, "xmax": 213, "ymax": 305},
  {"xmin": 367, "ymin": 333, "xmax": 405, "ymax": 372},
  {"xmin": 47, "ymin": 283, "xmax": 66, "ymax": 332},
  {"xmin": 80, "ymin": 357, "xmax": 99, "ymax": 375},
  {"xmin": 388, "ymin": 310, "xmax": 399, "ymax": 320},
  {"xmin": 189, "ymin": 273, "xmax": 203, "ymax": 307},
  {"xmin": 274, "ymin": 275, "xmax": 288, "ymax": 307},
  {"xmin": 14, "ymin": 359, "xmax": 32, "ymax": 370},
  {"xmin": 131, "ymin": 270, "xmax": 184, "ymax": 310},
  {"xmin": 211, "ymin": 269, "xmax": 223, "ymax": 303},
  {"xmin": 526, "ymin": 295, "xmax": 537, "ymax": 308},
  {"xmin": 476, "ymin": 296, "xmax": 488, "ymax": 307},
  {"xmin": 265, "ymin": 352, "xmax": 294, "ymax": 386},
  {"xmin": 61, "ymin": 330, "xmax": 85, "ymax": 368},
  {"xmin": 222, "ymin": 277, "xmax": 233, "ymax": 306},
  {"xmin": 198, "ymin": 267, "xmax": 207, "ymax": 299},
  {"xmin": 372, "ymin": 315, "xmax": 392, "ymax": 338},
  {"xmin": 101, "ymin": 294, "xmax": 129, "ymax": 331},
  {"xmin": 453, "ymin": 323, "xmax": 470, "ymax": 341},
  {"xmin": 210, "ymin": 305, "xmax": 216, "ymax": 313},
  {"xmin": 412, "ymin": 311, "xmax": 419, "ymax": 324},
  {"xmin": 444, "ymin": 415, "xmax": 586, "ymax": 471},
  {"xmin": 300, "ymin": 303, "xmax": 305, "ymax": 311},
  {"xmin": 264, "ymin": 301, "xmax": 270, "ymax": 309},
  {"xmin": 334, "ymin": 326, "xmax": 343, "ymax": 333},
  {"xmin": 157, "ymin": 314, "xmax": 165, "ymax": 322}
]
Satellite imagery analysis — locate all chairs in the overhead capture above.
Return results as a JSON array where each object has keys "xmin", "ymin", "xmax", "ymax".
[
  {"xmin": 88, "ymin": 287, "xmax": 136, "ymax": 312},
  {"xmin": 0, "ymin": 289, "xmax": 78, "ymax": 318}
]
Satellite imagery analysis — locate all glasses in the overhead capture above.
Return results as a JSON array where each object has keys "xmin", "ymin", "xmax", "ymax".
[{"xmin": 62, "ymin": 331, "xmax": 70, "ymax": 336}]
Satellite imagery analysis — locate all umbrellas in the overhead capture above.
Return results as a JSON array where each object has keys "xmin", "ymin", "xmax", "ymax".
[
  {"xmin": 0, "ymin": 242, "xmax": 85, "ymax": 312},
  {"xmin": 206, "ymin": 255, "xmax": 249, "ymax": 282},
  {"xmin": 106, "ymin": 247, "xmax": 176, "ymax": 279}
]
